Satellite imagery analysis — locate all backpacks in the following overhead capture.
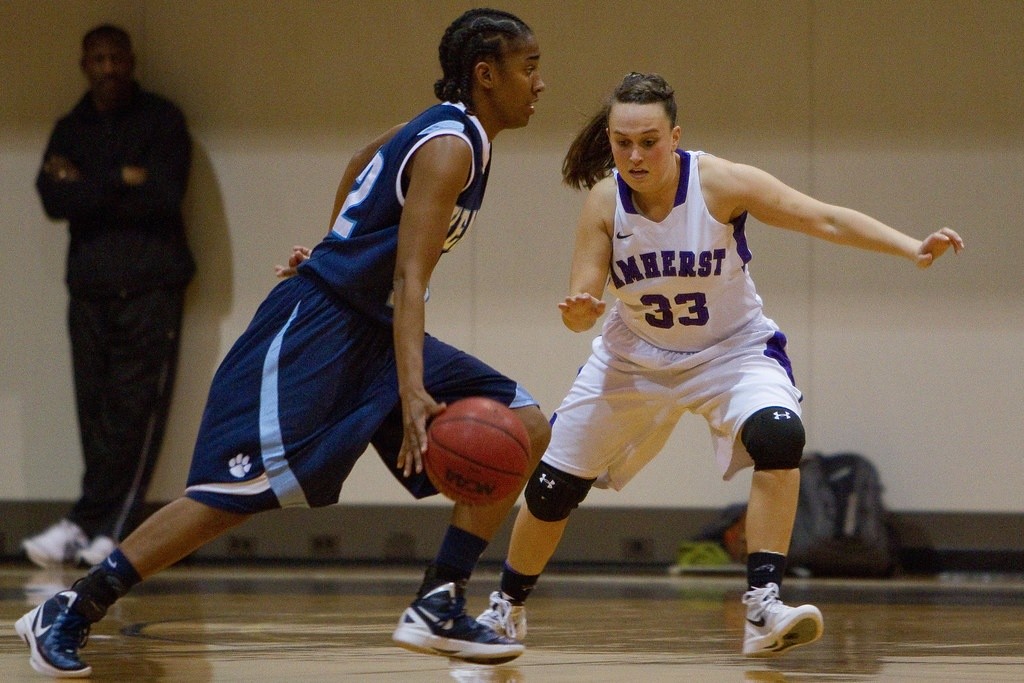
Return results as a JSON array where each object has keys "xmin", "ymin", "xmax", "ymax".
[{"xmin": 796, "ymin": 452, "xmax": 889, "ymax": 574}]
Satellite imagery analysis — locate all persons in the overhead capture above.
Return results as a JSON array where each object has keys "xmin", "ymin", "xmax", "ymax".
[
  {"xmin": 14, "ymin": 24, "xmax": 193, "ymax": 572},
  {"xmin": 14, "ymin": 6, "xmax": 549, "ymax": 677},
  {"xmin": 471, "ymin": 69, "xmax": 965, "ymax": 659}
]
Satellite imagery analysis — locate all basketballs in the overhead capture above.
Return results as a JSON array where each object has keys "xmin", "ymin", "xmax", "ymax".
[{"xmin": 423, "ymin": 397, "xmax": 532, "ymax": 507}]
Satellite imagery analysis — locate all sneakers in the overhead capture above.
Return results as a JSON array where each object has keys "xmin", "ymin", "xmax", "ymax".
[
  {"xmin": 13, "ymin": 590, "xmax": 106, "ymax": 678},
  {"xmin": 475, "ymin": 590, "xmax": 528, "ymax": 641},
  {"xmin": 391, "ymin": 579, "xmax": 525, "ymax": 659},
  {"xmin": 741, "ymin": 583, "xmax": 826, "ymax": 658},
  {"xmin": 21, "ymin": 518, "xmax": 89, "ymax": 569},
  {"xmin": 74, "ymin": 537, "xmax": 116, "ymax": 566}
]
